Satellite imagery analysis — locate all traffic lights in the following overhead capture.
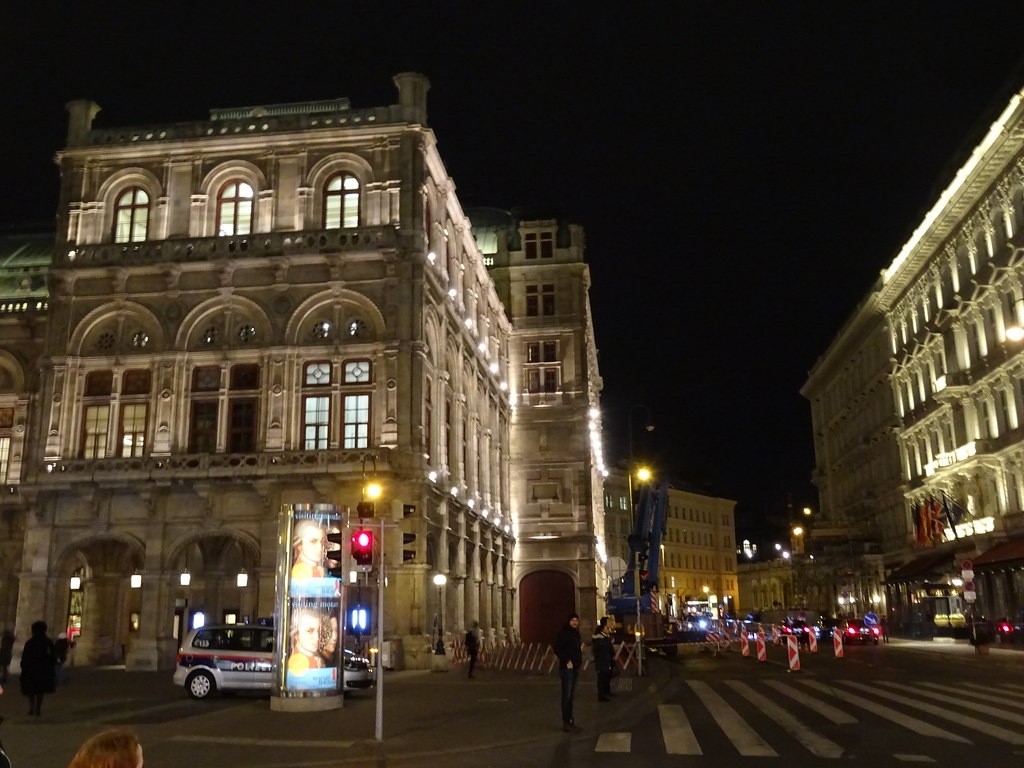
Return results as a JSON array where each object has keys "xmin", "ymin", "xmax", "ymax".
[
  {"xmin": 392, "ymin": 527, "xmax": 417, "ymax": 569},
  {"xmin": 392, "ymin": 499, "xmax": 416, "ymax": 522},
  {"xmin": 636, "ymin": 566, "xmax": 651, "ymax": 597},
  {"xmin": 327, "ymin": 530, "xmax": 343, "ymax": 582},
  {"xmin": 357, "ymin": 502, "xmax": 374, "ymax": 518},
  {"xmin": 635, "ymin": 550, "xmax": 650, "ymax": 565},
  {"xmin": 357, "ymin": 530, "xmax": 373, "ymax": 565}
]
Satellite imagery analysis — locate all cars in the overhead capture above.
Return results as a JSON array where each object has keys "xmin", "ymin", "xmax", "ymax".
[
  {"xmin": 175, "ymin": 622, "xmax": 377, "ymax": 702},
  {"xmin": 669, "ymin": 610, "xmax": 880, "ymax": 647},
  {"xmin": 971, "ymin": 617, "xmax": 1024, "ymax": 646}
]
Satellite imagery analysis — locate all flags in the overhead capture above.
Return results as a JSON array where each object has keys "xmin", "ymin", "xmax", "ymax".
[{"xmin": 910, "ymin": 494, "xmax": 947, "ymax": 547}]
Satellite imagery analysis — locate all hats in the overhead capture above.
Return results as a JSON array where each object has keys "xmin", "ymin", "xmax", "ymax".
[{"xmin": 568, "ymin": 614, "xmax": 578, "ymax": 622}]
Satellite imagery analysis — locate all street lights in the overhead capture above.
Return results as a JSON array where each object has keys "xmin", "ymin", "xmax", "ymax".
[
  {"xmin": 432, "ymin": 574, "xmax": 447, "ymax": 656},
  {"xmin": 356, "ymin": 453, "xmax": 383, "ymax": 655},
  {"xmin": 628, "ymin": 464, "xmax": 655, "ymax": 676}
]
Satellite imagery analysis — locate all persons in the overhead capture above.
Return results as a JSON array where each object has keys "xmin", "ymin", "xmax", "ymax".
[
  {"xmin": 288, "ymin": 606, "xmax": 338, "ymax": 668},
  {"xmin": 20, "ymin": 620, "xmax": 56, "ymax": 717},
  {"xmin": 0, "ymin": 628, "xmax": 15, "ymax": 682},
  {"xmin": 551, "ymin": 614, "xmax": 588, "ymax": 733},
  {"xmin": 434, "ymin": 631, "xmax": 446, "ymax": 656},
  {"xmin": 686, "ymin": 612, "xmax": 695, "ymax": 622},
  {"xmin": 880, "ymin": 614, "xmax": 889, "ymax": 643},
  {"xmin": 773, "ymin": 599, "xmax": 783, "ymax": 610},
  {"xmin": 464, "ymin": 621, "xmax": 479, "ymax": 678},
  {"xmin": 781, "ymin": 618, "xmax": 810, "ymax": 653},
  {"xmin": 649, "ymin": 583, "xmax": 657, "ymax": 592},
  {"xmin": 592, "ymin": 624, "xmax": 613, "ymax": 702},
  {"xmin": 291, "ymin": 518, "xmax": 334, "ymax": 579},
  {"xmin": 600, "ymin": 617, "xmax": 617, "ymax": 697},
  {"xmin": 68, "ymin": 724, "xmax": 144, "ymax": 768}
]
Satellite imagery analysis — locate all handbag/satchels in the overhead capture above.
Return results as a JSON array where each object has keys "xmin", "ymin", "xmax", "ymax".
[{"xmin": 611, "ymin": 657, "xmax": 620, "ymax": 677}]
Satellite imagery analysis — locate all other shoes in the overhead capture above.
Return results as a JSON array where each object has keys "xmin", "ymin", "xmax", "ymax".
[
  {"xmin": 598, "ymin": 697, "xmax": 610, "ymax": 702},
  {"xmin": 27, "ymin": 708, "xmax": 34, "ymax": 716},
  {"xmin": 564, "ymin": 724, "xmax": 583, "ymax": 732},
  {"xmin": 34, "ymin": 710, "xmax": 41, "ymax": 716},
  {"xmin": 606, "ymin": 692, "xmax": 618, "ymax": 697}
]
